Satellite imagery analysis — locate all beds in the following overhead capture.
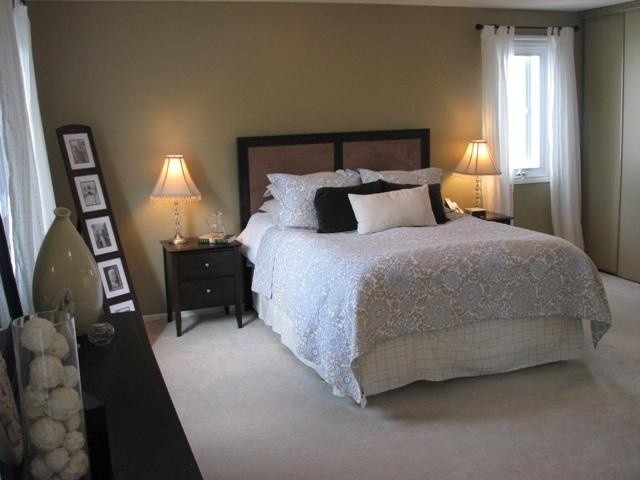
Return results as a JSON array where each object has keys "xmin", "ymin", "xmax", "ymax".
[{"xmin": 233, "ymin": 126, "xmax": 612, "ymax": 407}]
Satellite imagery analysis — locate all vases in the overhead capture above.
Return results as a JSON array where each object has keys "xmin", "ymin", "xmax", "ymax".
[{"xmin": 32, "ymin": 207, "xmax": 106, "ymax": 337}]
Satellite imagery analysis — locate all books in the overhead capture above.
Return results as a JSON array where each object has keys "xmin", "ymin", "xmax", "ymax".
[{"xmin": 197, "ymin": 232, "xmax": 237, "ymax": 244}]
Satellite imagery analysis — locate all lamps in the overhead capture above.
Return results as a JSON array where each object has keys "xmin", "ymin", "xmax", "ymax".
[
  {"xmin": 149, "ymin": 153, "xmax": 203, "ymax": 244},
  {"xmin": 452, "ymin": 139, "xmax": 502, "ymax": 212}
]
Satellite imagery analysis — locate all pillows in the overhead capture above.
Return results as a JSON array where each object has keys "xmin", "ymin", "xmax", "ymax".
[
  {"xmin": 264, "ymin": 170, "xmax": 360, "ymax": 230},
  {"xmin": 381, "ymin": 181, "xmax": 449, "ymax": 224},
  {"xmin": 314, "ymin": 179, "xmax": 387, "ymax": 232},
  {"xmin": 347, "ymin": 184, "xmax": 437, "ymax": 234},
  {"xmin": 359, "ymin": 165, "xmax": 450, "ymax": 219}
]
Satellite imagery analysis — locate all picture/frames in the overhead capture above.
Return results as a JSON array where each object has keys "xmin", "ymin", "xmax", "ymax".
[{"xmin": 55, "ymin": 123, "xmax": 144, "ymax": 328}]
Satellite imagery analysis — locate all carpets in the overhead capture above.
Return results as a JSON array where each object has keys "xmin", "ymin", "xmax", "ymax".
[{"xmin": 141, "ymin": 252, "xmax": 639, "ymax": 480}]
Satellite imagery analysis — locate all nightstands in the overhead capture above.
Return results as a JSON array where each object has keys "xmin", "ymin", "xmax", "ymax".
[
  {"xmin": 158, "ymin": 234, "xmax": 245, "ymax": 338},
  {"xmin": 466, "ymin": 210, "xmax": 516, "ymax": 226}
]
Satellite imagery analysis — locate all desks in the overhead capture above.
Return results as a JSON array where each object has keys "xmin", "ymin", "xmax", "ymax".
[{"xmin": 2, "ymin": 312, "xmax": 203, "ymax": 478}]
